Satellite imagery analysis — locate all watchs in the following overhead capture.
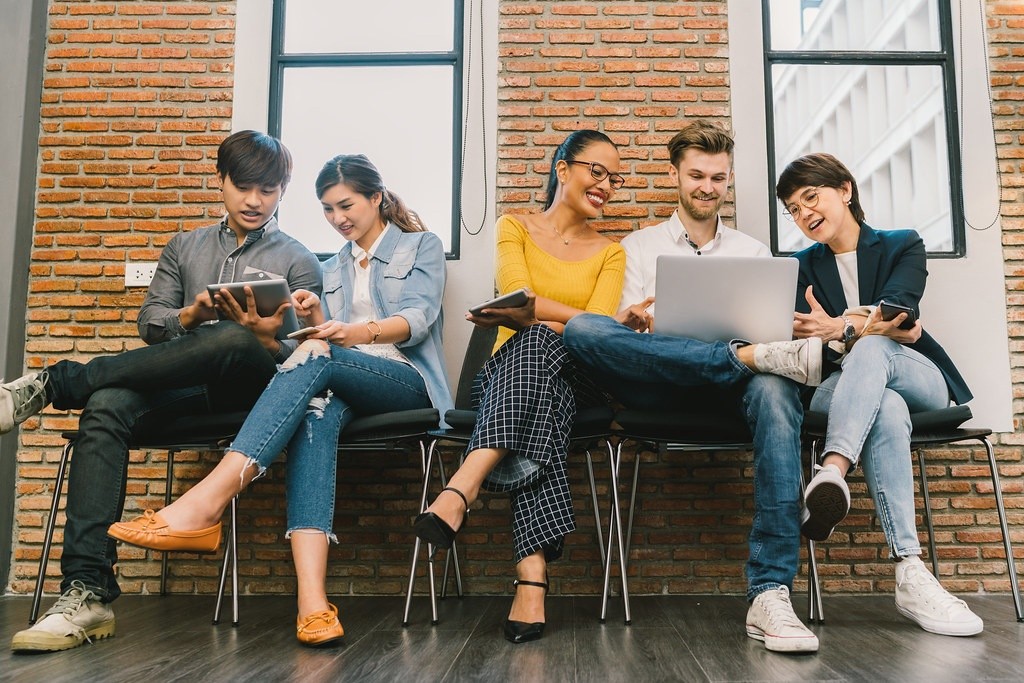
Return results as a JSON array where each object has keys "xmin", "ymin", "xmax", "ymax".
[{"xmin": 838, "ymin": 315, "xmax": 855, "ymax": 343}]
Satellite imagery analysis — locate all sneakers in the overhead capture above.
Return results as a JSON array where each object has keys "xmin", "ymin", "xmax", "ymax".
[
  {"xmin": 754, "ymin": 337, "xmax": 823, "ymax": 386},
  {"xmin": 11, "ymin": 580, "xmax": 114, "ymax": 651},
  {"xmin": 0, "ymin": 372, "xmax": 49, "ymax": 434},
  {"xmin": 744, "ymin": 585, "xmax": 819, "ymax": 652},
  {"xmin": 801, "ymin": 464, "xmax": 852, "ymax": 543},
  {"xmin": 894, "ymin": 557, "xmax": 984, "ymax": 636}
]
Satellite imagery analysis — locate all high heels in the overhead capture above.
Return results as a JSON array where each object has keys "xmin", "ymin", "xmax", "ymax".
[
  {"xmin": 413, "ymin": 488, "xmax": 471, "ymax": 558},
  {"xmin": 504, "ymin": 570, "xmax": 549, "ymax": 643}
]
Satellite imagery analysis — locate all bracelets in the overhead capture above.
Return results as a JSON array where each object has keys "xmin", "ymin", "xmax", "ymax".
[{"xmin": 367, "ymin": 321, "xmax": 382, "ymax": 344}]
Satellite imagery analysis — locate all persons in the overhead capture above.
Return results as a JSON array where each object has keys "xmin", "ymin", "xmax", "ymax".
[
  {"xmin": 414, "ymin": 128, "xmax": 655, "ymax": 644},
  {"xmin": 774, "ymin": 152, "xmax": 984, "ymax": 637},
  {"xmin": 106, "ymin": 152, "xmax": 456, "ymax": 646},
  {"xmin": 562, "ymin": 120, "xmax": 823, "ymax": 653},
  {"xmin": 0, "ymin": 133, "xmax": 323, "ymax": 650}
]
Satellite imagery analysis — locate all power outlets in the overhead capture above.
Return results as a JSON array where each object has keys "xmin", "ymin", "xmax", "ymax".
[{"xmin": 124, "ymin": 262, "xmax": 158, "ymax": 287}]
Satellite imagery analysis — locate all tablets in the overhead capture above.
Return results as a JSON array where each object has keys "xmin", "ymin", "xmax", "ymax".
[
  {"xmin": 469, "ymin": 288, "xmax": 529, "ymax": 317},
  {"xmin": 205, "ymin": 279, "xmax": 300, "ymax": 342}
]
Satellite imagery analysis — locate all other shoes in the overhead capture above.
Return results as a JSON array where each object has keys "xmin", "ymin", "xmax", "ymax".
[
  {"xmin": 296, "ymin": 603, "xmax": 344, "ymax": 646},
  {"xmin": 106, "ymin": 508, "xmax": 222, "ymax": 555}
]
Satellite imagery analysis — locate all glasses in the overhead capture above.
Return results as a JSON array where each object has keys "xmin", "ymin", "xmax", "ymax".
[
  {"xmin": 782, "ymin": 184, "xmax": 824, "ymax": 222},
  {"xmin": 555, "ymin": 159, "xmax": 625, "ymax": 190}
]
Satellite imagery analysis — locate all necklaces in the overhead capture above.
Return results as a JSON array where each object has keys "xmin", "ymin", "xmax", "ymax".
[{"xmin": 553, "ymin": 227, "xmax": 581, "ymax": 245}]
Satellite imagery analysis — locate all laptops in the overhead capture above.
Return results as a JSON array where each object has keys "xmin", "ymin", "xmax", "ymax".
[{"xmin": 654, "ymin": 254, "xmax": 799, "ymax": 342}]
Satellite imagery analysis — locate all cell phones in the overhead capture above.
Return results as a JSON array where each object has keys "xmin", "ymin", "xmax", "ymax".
[
  {"xmin": 880, "ymin": 302, "xmax": 916, "ymax": 330},
  {"xmin": 287, "ymin": 328, "xmax": 325, "ymax": 341}
]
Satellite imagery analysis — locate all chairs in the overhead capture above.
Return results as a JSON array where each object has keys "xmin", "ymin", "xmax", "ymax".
[{"xmin": 28, "ymin": 324, "xmax": 1024, "ymax": 629}]
